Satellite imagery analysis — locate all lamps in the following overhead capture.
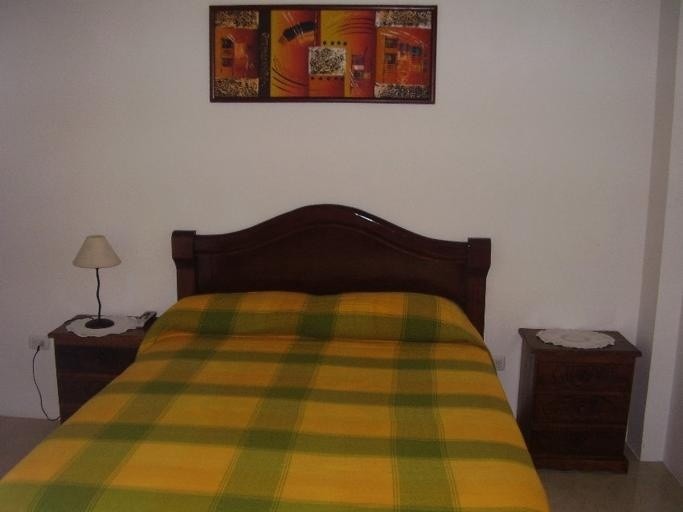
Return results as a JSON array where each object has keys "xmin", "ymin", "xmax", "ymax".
[{"xmin": 72, "ymin": 234, "xmax": 121, "ymax": 330}]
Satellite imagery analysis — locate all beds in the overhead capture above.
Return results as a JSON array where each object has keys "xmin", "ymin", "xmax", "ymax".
[{"xmin": 0, "ymin": 202, "xmax": 548, "ymax": 511}]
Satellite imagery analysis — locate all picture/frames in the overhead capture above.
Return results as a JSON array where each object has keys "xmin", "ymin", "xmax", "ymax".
[{"xmin": 207, "ymin": 3, "xmax": 439, "ymax": 107}]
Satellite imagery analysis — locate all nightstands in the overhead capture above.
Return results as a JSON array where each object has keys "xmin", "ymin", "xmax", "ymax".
[
  {"xmin": 516, "ymin": 327, "xmax": 642, "ymax": 476},
  {"xmin": 45, "ymin": 313, "xmax": 160, "ymax": 424}
]
